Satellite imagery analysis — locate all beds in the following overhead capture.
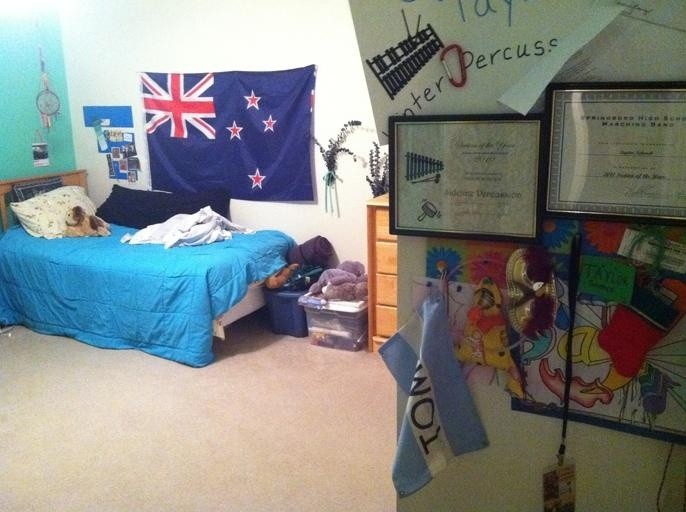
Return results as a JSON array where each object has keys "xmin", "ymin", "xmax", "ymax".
[{"xmin": 0, "ymin": 168, "xmax": 297, "ymax": 368}]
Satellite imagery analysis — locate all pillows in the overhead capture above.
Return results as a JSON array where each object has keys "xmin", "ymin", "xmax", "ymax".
[
  {"xmin": 169, "ymin": 179, "xmax": 232, "ymax": 224},
  {"xmin": 10, "ymin": 184, "xmax": 110, "ymax": 241},
  {"xmin": 96, "ymin": 184, "xmax": 170, "ymax": 230}
]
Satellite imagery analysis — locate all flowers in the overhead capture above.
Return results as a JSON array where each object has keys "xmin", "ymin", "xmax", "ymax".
[
  {"xmin": 312, "ymin": 120, "xmax": 364, "ymax": 214},
  {"xmin": 366, "ymin": 141, "xmax": 389, "ymax": 197}
]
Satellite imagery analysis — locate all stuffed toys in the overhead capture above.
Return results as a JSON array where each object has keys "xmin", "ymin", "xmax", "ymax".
[
  {"xmin": 314, "ymin": 281, "xmax": 368, "ymax": 301},
  {"xmin": 65, "ymin": 206, "xmax": 112, "ymax": 236},
  {"xmin": 265, "ymin": 263, "xmax": 329, "ymax": 292},
  {"xmin": 309, "ymin": 261, "xmax": 367, "ymax": 293}
]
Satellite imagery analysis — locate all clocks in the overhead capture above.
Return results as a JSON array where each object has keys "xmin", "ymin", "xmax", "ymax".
[{"xmin": 37, "ymin": 60, "xmax": 62, "ymax": 128}]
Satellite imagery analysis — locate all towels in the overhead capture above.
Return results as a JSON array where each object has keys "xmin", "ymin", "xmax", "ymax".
[{"xmin": 378, "ymin": 291, "xmax": 490, "ymax": 494}]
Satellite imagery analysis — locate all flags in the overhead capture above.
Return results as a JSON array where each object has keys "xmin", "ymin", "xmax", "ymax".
[{"xmin": 138, "ymin": 64, "xmax": 314, "ymax": 203}]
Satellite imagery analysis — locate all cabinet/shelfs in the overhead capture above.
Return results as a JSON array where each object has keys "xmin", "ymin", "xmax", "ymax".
[{"xmin": 367, "ymin": 191, "xmax": 399, "ymax": 353}]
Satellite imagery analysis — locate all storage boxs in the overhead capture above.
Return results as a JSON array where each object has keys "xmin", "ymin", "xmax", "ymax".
[
  {"xmin": 261, "ymin": 284, "xmax": 308, "ymax": 335},
  {"xmin": 300, "ymin": 293, "xmax": 366, "ymax": 351}
]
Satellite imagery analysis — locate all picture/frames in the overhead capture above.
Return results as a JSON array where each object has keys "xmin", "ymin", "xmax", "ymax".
[
  {"xmin": 389, "ymin": 113, "xmax": 546, "ymax": 245},
  {"xmin": 541, "ymin": 81, "xmax": 685, "ymax": 226}
]
